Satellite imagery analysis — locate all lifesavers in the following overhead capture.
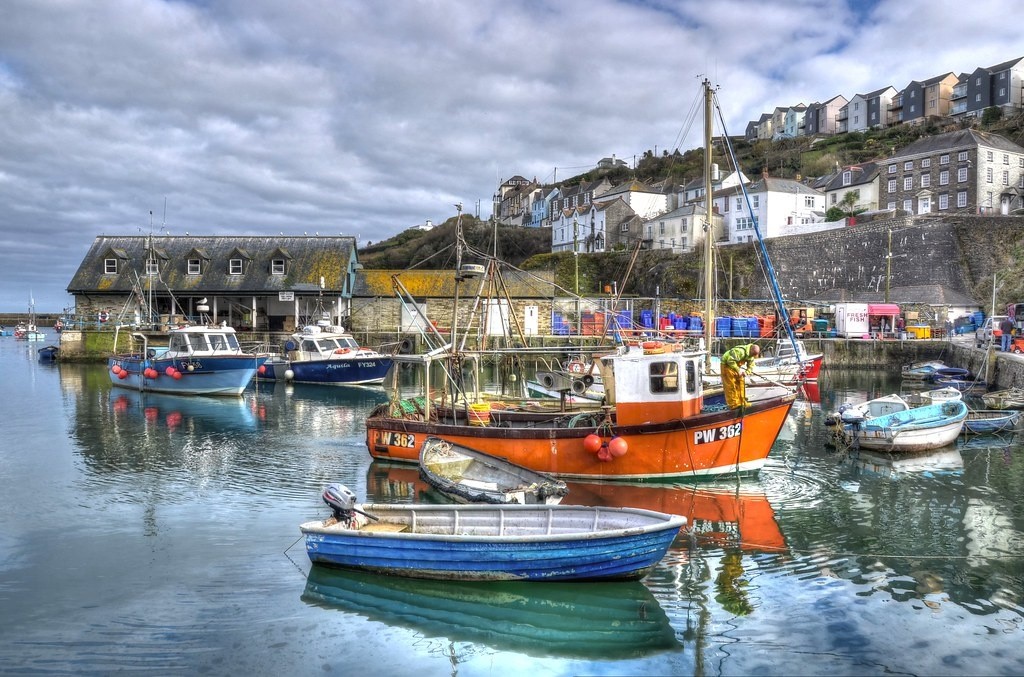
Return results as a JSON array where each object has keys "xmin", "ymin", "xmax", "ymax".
[
  {"xmin": 98, "ymin": 311, "xmax": 110, "ymax": 321},
  {"xmin": 358, "ymin": 347, "xmax": 368, "ymax": 350},
  {"xmin": 335, "ymin": 348, "xmax": 350, "ymax": 353},
  {"xmin": 569, "ymin": 361, "xmax": 585, "ymax": 373}
]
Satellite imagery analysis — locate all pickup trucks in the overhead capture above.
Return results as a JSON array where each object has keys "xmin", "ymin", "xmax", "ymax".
[{"xmin": 975, "ymin": 314, "xmax": 1010, "ymax": 350}]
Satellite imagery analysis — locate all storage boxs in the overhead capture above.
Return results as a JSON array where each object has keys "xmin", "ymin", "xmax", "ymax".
[
  {"xmin": 905, "ymin": 326, "xmax": 931, "ymax": 339},
  {"xmin": 553, "ymin": 308, "xmax": 827, "ymax": 338},
  {"xmin": 974, "ymin": 312, "xmax": 984, "ymax": 330},
  {"xmin": 283, "ymin": 316, "xmax": 295, "ymax": 331}
]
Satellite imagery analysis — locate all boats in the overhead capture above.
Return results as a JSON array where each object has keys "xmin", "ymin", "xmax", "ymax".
[
  {"xmin": 108, "ymin": 208, "xmax": 273, "ymax": 395},
  {"xmin": 296, "ymin": 482, "xmax": 690, "ymax": 582},
  {"xmin": 108, "ymin": 387, "xmax": 258, "ymax": 433},
  {"xmin": 0, "ymin": 290, "xmax": 68, "ymax": 341},
  {"xmin": 37, "ymin": 344, "xmax": 59, "ymax": 362},
  {"xmin": 251, "ymin": 288, "xmax": 394, "ymax": 386},
  {"xmin": 842, "ymin": 440, "xmax": 965, "ymax": 482},
  {"xmin": 300, "ymin": 563, "xmax": 686, "ymax": 660},
  {"xmin": 418, "ymin": 432, "xmax": 570, "ymax": 511},
  {"xmin": 826, "ymin": 358, "xmax": 1024, "ymax": 451},
  {"xmin": 251, "ymin": 381, "xmax": 392, "ymax": 405}
]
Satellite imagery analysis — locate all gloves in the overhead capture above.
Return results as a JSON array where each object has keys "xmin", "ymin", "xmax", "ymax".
[{"xmin": 739, "ymin": 369, "xmax": 747, "ymax": 378}]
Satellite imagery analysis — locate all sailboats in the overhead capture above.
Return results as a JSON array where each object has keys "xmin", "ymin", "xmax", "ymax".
[
  {"xmin": 363, "ymin": 459, "xmax": 792, "ymax": 677},
  {"xmin": 364, "ymin": 70, "xmax": 822, "ymax": 484}
]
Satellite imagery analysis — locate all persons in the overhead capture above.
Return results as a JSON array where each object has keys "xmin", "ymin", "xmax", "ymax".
[
  {"xmin": 869, "ymin": 315, "xmax": 892, "ymax": 332},
  {"xmin": 1008, "ymin": 317, "xmax": 1016, "ymax": 323},
  {"xmin": 720, "ymin": 343, "xmax": 760, "ymax": 409},
  {"xmin": 1001, "ymin": 318, "xmax": 1013, "ymax": 352}
]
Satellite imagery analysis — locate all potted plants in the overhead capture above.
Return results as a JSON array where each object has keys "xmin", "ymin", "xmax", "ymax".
[{"xmin": 844, "ymin": 191, "xmax": 859, "ymax": 225}]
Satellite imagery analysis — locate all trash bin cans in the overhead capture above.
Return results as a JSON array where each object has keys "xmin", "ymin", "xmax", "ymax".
[
  {"xmin": 811, "ymin": 320, "xmax": 828, "ymax": 339},
  {"xmin": 717, "ymin": 315, "xmax": 775, "ymax": 337}
]
